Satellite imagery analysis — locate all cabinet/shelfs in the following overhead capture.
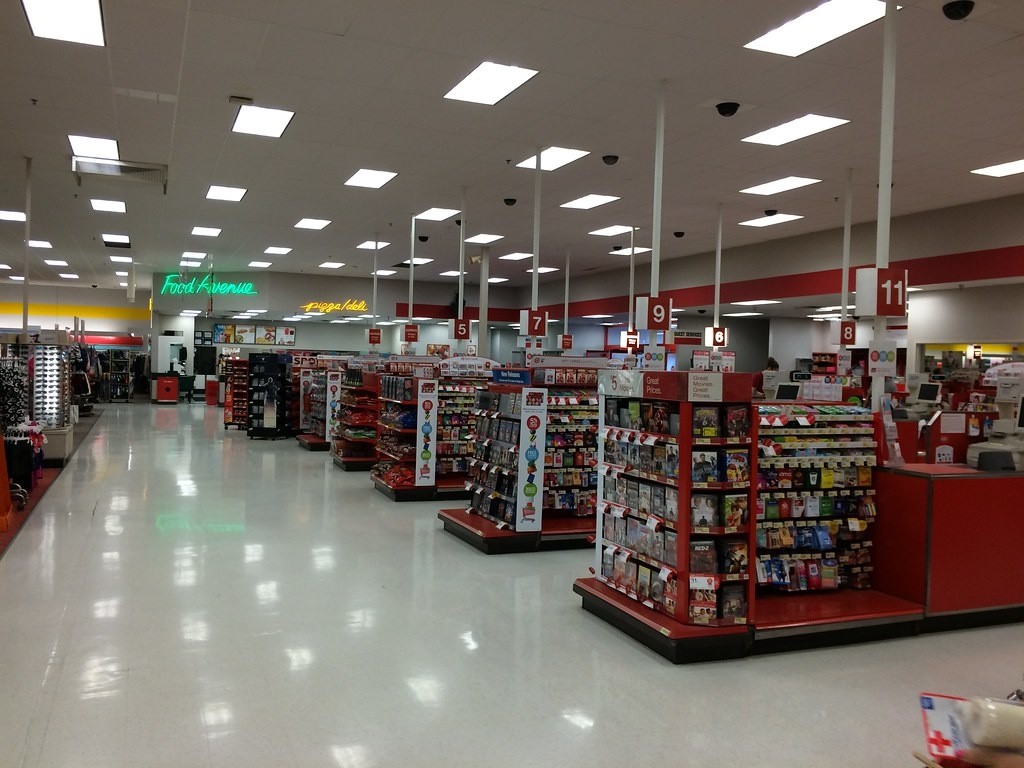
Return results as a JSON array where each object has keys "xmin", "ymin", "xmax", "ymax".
[{"xmin": 1, "ymin": 327, "xmax": 1024, "ymax": 664}]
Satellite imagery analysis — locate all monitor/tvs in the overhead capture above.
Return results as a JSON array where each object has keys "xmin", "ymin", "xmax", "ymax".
[
  {"xmin": 1015, "ymin": 394, "xmax": 1024, "ymax": 430},
  {"xmin": 774, "ymin": 382, "xmax": 803, "ymax": 401},
  {"xmin": 916, "ymin": 383, "xmax": 942, "ymax": 403}
]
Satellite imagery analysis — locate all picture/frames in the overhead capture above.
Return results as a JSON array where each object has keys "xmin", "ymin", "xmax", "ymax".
[
  {"xmin": 276, "ymin": 326, "xmax": 296, "ymax": 346},
  {"xmin": 214, "ymin": 324, "xmax": 235, "ymax": 344},
  {"xmin": 235, "ymin": 324, "xmax": 256, "ymax": 344},
  {"xmin": 255, "ymin": 325, "xmax": 276, "ymax": 344}
]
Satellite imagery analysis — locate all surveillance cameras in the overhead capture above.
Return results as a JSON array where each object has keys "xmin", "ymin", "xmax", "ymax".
[
  {"xmin": 715, "ymin": 102, "xmax": 740, "ymax": 117},
  {"xmin": 613, "ymin": 246, "xmax": 622, "ymax": 251},
  {"xmin": 943, "ymin": 0, "xmax": 975, "ymax": 20},
  {"xmin": 419, "ymin": 236, "xmax": 428, "ymax": 242},
  {"xmin": 602, "ymin": 155, "xmax": 618, "ymax": 165},
  {"xmin": 698, "ymin": 310, "xmax": 705, "ymax": 313},
  {"xmin": 765, "ymin": 210, "xmax": 777, "ymax": 216},
  {"xmin": 504, "ymin": 199, "xmax": 517, "ymax": 206},
  {"xmin": 456, "ymin": 220, "xmax": 461, "ymax": 226},
  {"xmin": 852, "ymin": 316, "xmax": 860, "ymax": 319},
  {"xmin": 92, "ymin": 285, "xmax": 97, "ymax": 288},
  {"xmin": 674, "ymin": 232, "xmax": 684, "ymax": 237}
]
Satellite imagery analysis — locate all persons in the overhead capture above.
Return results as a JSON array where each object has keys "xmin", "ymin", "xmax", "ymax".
[
  {"xmin": 667, "ymin": 446, "xmax": 678, "ymax": 464},
  {"xmin": 724, "ymin": 598, "xmax": 743, "ymax": 617},
  {"xmin": 655, "ymin": 455, "xmax": 663, "ymax": 470},
  {"xmin": 973, "ymin": 395, "xmax": 982, "ymax": 412},
  {"xmin": 694, "ymin": 453, "xmax": 715, "ymax": 482},
  {"xmin": 752, "ymin": 357, "xmax": 779, "ymax": 398},
  {"xmin": 735, "ymin": 497, "xmax": 748, "ymax": 524},
  {"xmin": 691, "ymin": 496, "xmax": 715, "ymax": 509}
]
